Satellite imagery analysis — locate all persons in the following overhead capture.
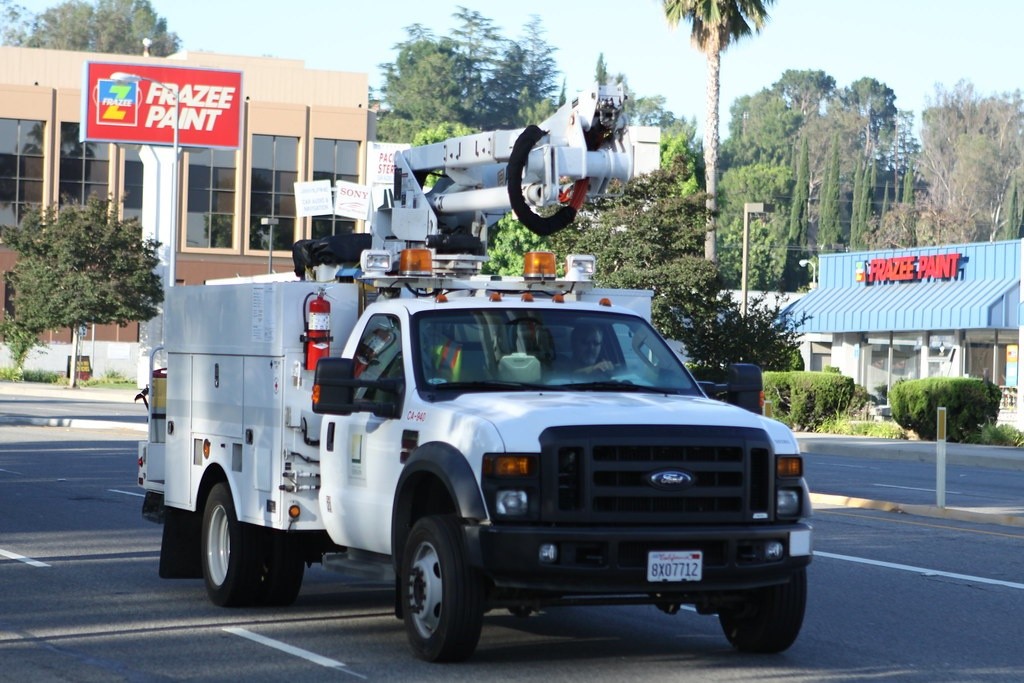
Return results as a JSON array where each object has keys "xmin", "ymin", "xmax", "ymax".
[{"xmin": 555, "ymin": 323, "xmax": 621, "ymax": 374}]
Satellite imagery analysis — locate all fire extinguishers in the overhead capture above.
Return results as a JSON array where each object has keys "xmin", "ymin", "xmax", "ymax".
[{"xmin": 301, "ymin": 285, "xmax": 338, "ymax": 371}]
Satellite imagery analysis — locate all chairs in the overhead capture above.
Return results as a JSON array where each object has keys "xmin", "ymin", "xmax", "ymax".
[{"xmin": 499, "ymin": 317, "xmax": 555, "ymax": 362}]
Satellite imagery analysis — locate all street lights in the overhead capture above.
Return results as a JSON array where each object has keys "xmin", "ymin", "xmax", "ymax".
[{"xmin": 741, "ymin": 202, "xmax": 775, "ymax": 319}]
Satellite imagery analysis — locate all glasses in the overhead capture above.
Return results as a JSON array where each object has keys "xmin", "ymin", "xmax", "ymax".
[{"xmin": 579, "ymin": 339, "xmax": 602, "ymax": 346}]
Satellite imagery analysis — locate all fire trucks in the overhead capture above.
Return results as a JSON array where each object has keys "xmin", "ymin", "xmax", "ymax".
[{"xmin": 137, "ymin": 82, "xmax": 813, "ymax": 662}]
